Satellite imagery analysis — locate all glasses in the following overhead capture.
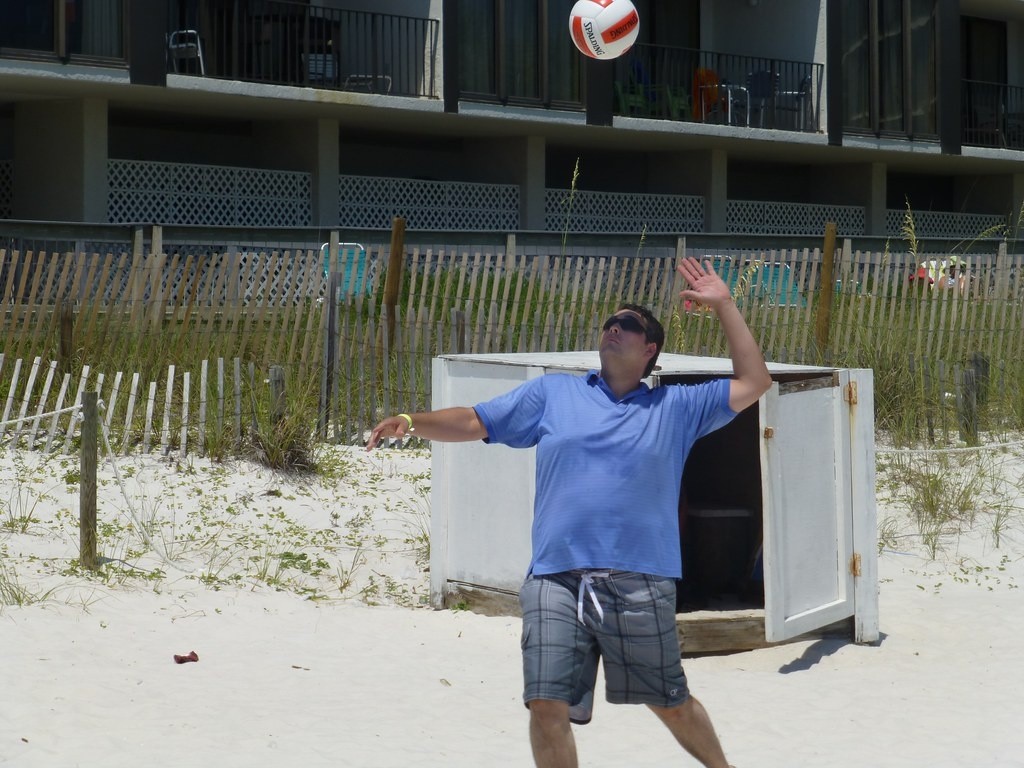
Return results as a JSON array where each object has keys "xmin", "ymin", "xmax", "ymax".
[{"xmin": 603, "ymin": 317, "xmax": 652, "ymax": 344}]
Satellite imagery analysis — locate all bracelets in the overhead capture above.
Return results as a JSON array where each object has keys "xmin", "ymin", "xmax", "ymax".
[{"xmin": 397, "ymin": 414, "xmax": 415, "ymax": 432}]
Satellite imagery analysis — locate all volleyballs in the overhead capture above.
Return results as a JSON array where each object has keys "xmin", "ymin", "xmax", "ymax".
[{"xmin": 568, "ymin": 0, "xmax": 640, "ymax": 61}]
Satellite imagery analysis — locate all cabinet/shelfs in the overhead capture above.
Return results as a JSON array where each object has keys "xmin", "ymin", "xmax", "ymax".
[{"xmin": 430, "ymin": 349, "xmax": 881, "ymax": 654}]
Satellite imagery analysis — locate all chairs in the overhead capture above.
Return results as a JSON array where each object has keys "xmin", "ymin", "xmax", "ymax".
[
  {"xmin": 320, "ymin": 243, "xmax": 374, "ymax": 301},
  {"xmin": 614, "ymin": 49, "xmax": 811, "ymax": 131},
  {"xmin": 698, "ymin": 255, "xmax": 808, "ymax": 310},
  {"xmin": 165, "ymin": 28, "xmax": 205, "ymax": 76},
  {"xmin": 302, "ymin": 52, "xmax": 392, "ymax": 93},
  {"xmin": 963, "ymin": 103, "xmax": 1024, "ymax": 151}
]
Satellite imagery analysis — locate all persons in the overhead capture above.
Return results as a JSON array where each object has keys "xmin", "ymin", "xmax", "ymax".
[
  {"xmin": 936, "ymin": 256, "xmax": 967, "ymax": 296},
  {"xmin": 909, "ymin": 268, "xmax": 935, "ymax": 286},
  {"xmin": 366, "ymin": 257, "xmax": 772, "ymax": 768}
]
